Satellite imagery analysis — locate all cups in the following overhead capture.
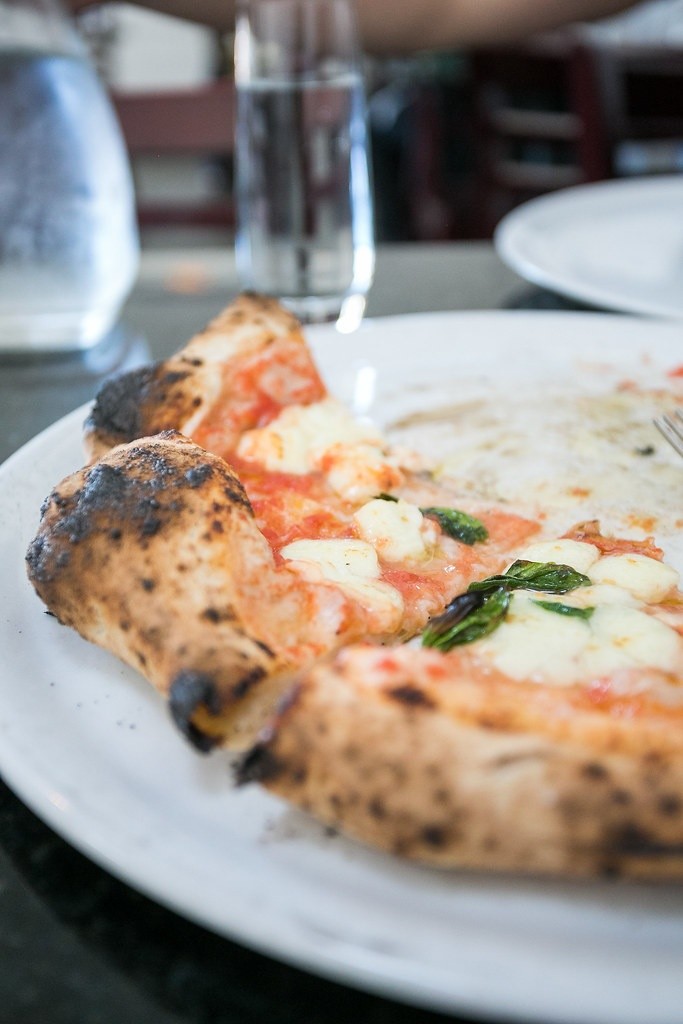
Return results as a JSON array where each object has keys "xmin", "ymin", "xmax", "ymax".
[{"xmin": 231, "ymin": 0, "xmax": 377, "ymax": 323}]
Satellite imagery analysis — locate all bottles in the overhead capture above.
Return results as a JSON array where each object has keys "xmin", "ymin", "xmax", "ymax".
[{"xmin": 0, "ymin": 0, "xmax": 140, "ymax": 356}]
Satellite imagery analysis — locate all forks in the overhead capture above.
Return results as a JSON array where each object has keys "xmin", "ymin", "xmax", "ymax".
[{"xmin": 653, "ymin": 408, "xmax": 683, "ymax": 458}]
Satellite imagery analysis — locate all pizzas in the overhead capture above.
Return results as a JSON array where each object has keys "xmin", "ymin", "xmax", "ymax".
[{"xmin": 20, "ymin": 291, "xmax": 683, "ymax": 883}]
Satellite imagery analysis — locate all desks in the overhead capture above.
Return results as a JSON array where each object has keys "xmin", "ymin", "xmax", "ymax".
[{"xmin": 0, "ymin": 237, "xmax": 631, "ymax": 1024}]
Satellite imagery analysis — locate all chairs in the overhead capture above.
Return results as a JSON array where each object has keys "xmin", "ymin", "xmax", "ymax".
[
  {"xmin": 454, "ymin": 34, "xmax": 618, "ymax": 241},
  {"xmin": 114, "ymin": 84, "xmax": 353, "ymax": 253}
]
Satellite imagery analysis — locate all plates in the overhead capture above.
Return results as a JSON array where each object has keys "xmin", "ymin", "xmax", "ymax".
[
  {"xmin": 0, "ymin": 309, "xmax": 683, "ymax": 1024},
  {"xmin": 493, "ymin": 174, "xmax": 683, "ymax": 320}
]
